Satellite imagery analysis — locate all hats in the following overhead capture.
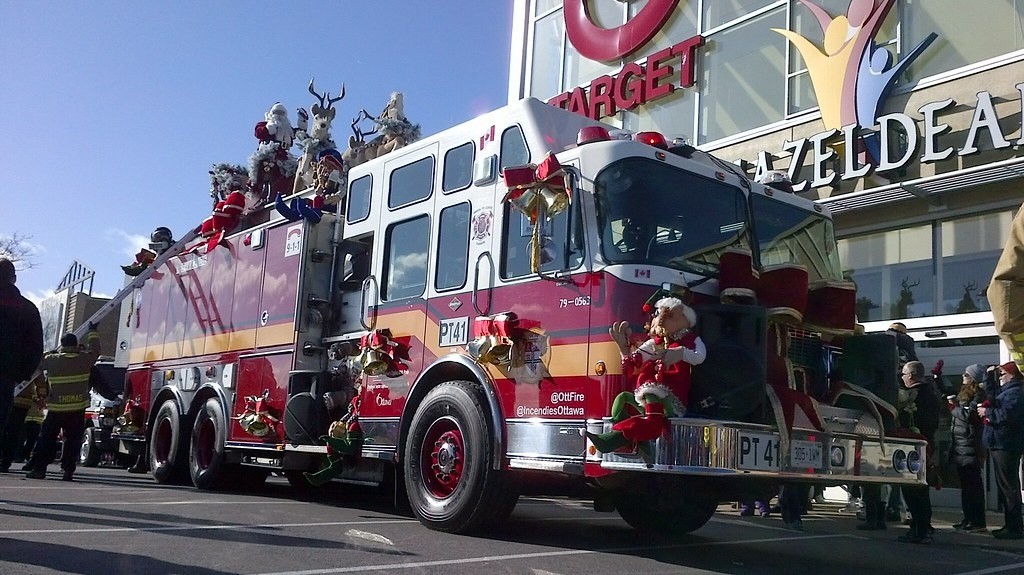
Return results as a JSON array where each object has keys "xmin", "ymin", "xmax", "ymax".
[
  {"xmin": 1000, "ymin": 361, "xmax": 1019, "ymax": 377},
  {"xmin": 966, "ymin": 364, "xmax": 985, "ymax": 383},
  {"xmin": 891, "ymin": 323, "xmax": 907, "ymax": 334}
]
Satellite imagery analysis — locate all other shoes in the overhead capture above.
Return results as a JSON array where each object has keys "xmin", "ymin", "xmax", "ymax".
[
  {"xmin": 63, "ymin": 471, "xmax": 73, "ymax": 481},
  {"xmin": 839, "ymin": 505, "xmax": 858, "ymax": 514},
  {"xmin": 783, "ymin": 520, "xmax": 805, "ymax": 531},
  {"xmin": 856, "ymin": 511, "xmax": 865, "ymax": 520},
  {"xmin": 811, "ymin": 494, "xmax": 828, "ymax": 504},
  {"xmin": 800, "ymin": 511, "xmax": 807, "ymax": 515},
  {"xmin": 22, "ymin": 460, "xmax": 34, "ymax": 471},
  {"xmin": 952, "ymin": 522, "xmax": 987, "ymax": 533},
  {"xmin": 992, "ymin": 527, "xmax": 1024, "ymax": 539},
  {"xmin": 762, "ymin": 512, "xmax": 769, "ymax": 517},
  {"xmin": 885, "ymin": 512, "xmax": 901, "ymax": 522},
  {"xmin": 128, "ymin": 464, "xmax": 147, "ymax": 474},
  {"xmin": 120, "ymin": 265, "xmax": 143, "ymax": 276},
  {"xmin": 770, "ymin": 507, "xmax": 782, "ymax": 513},
  {"xmin": 857, "ymin": 521, "xmax": 887, "ymax": 531},
  {"xmin": 807, "ymin": 503, "xmax": 813, "ymax": 511},
  {"xmin": 741, "ymin": 510, "xmax": 753, "ymax": 516},
  {"xmin": 903, "ymin": 519, "xmax": 912, "ymax": 525},
  {"xmin": 896, "ymin": 530, "xmax": 933, "ymax": 544},
  {"xmin": 26, "ymin": 471, "xmax": 46, "ymax": 479}
]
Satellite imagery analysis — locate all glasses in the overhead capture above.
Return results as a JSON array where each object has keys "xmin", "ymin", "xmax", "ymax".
[
  {"xmin": 999, "ymin": 373, "xmax": 1007, "ymax": 376},
  {"xmin": 901, "ymin": 373, "xmax": 907, "ymax": 376},
  {"xmin": 964, "ymin": 373, "xmax": 969, "ymax": 377}
]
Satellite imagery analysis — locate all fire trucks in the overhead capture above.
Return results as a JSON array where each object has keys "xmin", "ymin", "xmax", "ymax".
[{"xmin": 79, "ymin": 95, "xmax": 930, "ymax": 535}]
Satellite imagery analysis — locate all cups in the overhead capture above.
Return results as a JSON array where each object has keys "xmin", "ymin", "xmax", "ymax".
[
  {"xmin": 947, "ymin": 395, "xmax": 956, "ymax": 403},
  {"xmin": 977, "ymin": 404, "xmax": 982, "ymax": 407}
]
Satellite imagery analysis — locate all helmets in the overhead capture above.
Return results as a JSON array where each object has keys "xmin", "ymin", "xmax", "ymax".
[{"xmin": 151, "ymin": 227, "xmax": 172, "ymax": 240}]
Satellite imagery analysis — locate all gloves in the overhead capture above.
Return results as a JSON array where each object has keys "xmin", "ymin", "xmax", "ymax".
[
  {"xmin": 89, "ymin": 321, "xmax": 101, "ymax": 331},
  {"xmin": 947, "ymin": 401, "xmax": 958, "ymax": 410}
]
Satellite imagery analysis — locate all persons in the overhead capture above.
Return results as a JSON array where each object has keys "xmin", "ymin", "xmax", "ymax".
[
  {"xmin": 986, "ymin": 199, "xmax": 1024, "ymax": 376},
  {"xmin": 0, "ymin": 258, "xmax": 43, "ymax": 473},
  {"xmin": 303, "ymin": 373, "xmax": 364, "ymax": 489},
  {"xmin": 949, "ymin": 362, "xmax": 1024, "ymax": 541},
  {"xmin": 841, "ymin": 322, "xmax": 946, "ymax": 544},
  {"xmin": 734, "ymin": 476, "xmax": 830, "ymax": 522},
  {"xmin": 247, "ymin": 102, "xmax": 308, "ymax": 212},
  {"xmin": 14, "ymin": 322, "xmax": 102, "ymax": 481},
  {"xmin": 578, "ymin": 297, "xmax": 708, "ymax": 456}
]
